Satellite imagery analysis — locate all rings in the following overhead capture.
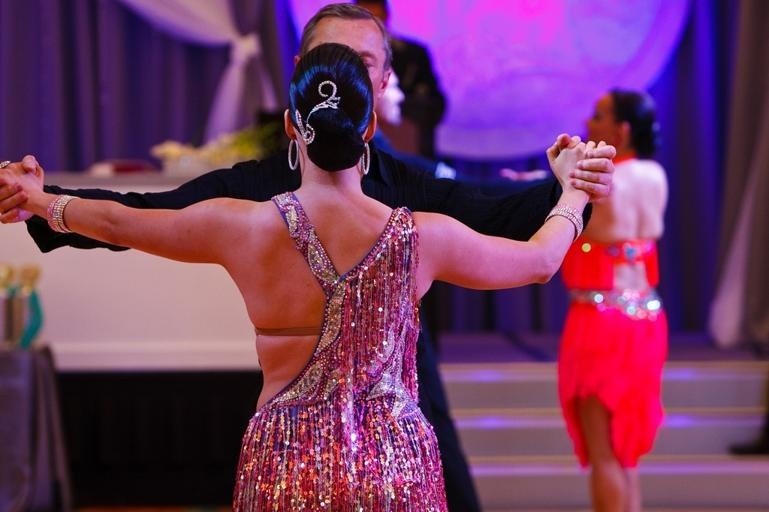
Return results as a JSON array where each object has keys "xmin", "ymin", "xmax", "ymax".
[{"xmin": 0, "ymin": 160, "xmax": 11, "ymax": 169}]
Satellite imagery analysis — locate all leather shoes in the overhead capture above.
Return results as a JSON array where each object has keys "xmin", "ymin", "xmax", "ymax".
[{"xmin": 728, "ymin": 441, "xmax": 768, "ymax": 456}]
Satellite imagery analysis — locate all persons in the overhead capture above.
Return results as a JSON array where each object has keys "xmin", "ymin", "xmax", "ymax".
[
  {"xmin": 352, "ymin": 0, "xmax": 447, "ymax": 160},
  {"xmin": 731, "ymin": 415, "xmax": 768, "ymax": 456},
  {"xmin": 552, "ymin": 84, "xmax": 668, "ymax": 512}
]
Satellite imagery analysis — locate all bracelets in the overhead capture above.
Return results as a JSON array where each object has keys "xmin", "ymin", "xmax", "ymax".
[
  {"xmin": 546, "ymin": 206, "xmax": 585, "ymax": 241},
  {"xmin": 48, "ymin": 196, "xmax": 77, "ymax": 233}
]
